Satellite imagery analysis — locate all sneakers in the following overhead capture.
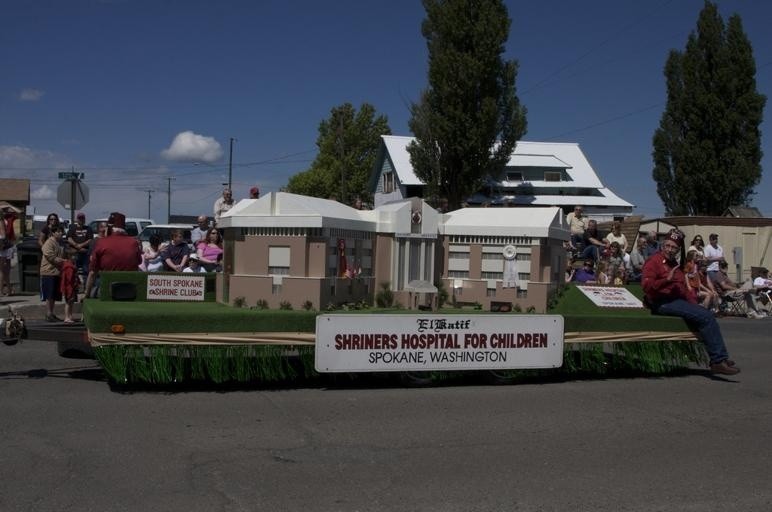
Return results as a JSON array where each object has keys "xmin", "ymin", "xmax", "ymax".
[
  {"xmin": 42, "ymin": 314, "xmax": 76, "ymax": 324},
  {"xmin": 714, "ymin": 307, "xmax": 769, "ymax": 319}
]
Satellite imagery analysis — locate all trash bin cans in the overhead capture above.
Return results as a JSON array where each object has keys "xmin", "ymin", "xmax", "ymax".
[{"xmin": 16, "ymin": 242, "xmax": 43, "ymax": 293}]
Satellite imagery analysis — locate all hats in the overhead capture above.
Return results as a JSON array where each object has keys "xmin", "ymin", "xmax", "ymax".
[
  {"xmin": 188, "ymin": 253, "xmax": 200, "ymax": 261},
  {"xmin": 663, "ymin": 226, "xmax": 685, "ymax": 248},
  {"xmin": 249, "ymin": 186, "xmax": 260, "ymax": 195},
  {"xmin": 106, "ymin": 212, "xmax": 126, "ymax": 229},
  {"xmin": 77, "ymin": 212, "xmax": 85, "ymax": 218}
]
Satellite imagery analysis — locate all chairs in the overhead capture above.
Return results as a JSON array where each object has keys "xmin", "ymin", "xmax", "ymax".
[{"xmin": 569, "ymin": 235, "xmax": 771, "ymax": 317}]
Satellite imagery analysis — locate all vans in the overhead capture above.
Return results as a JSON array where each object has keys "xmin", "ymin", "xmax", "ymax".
[{"xmin": 31, "ymin": 215, "xmax": 207, "ymax": 260}]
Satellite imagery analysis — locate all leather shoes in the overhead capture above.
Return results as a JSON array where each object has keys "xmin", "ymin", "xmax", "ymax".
[{"xmin": 709, "ymin": 359, "xmax": 740, "ymax": 377}]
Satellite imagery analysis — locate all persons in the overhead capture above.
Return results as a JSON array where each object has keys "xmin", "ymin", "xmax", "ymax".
[
  {"xmin": 683, "ymin": 233, "xmax": 772, "ymax": 320},
  {"xmin": 214, "ymin": 188, "xmax": 236, "ymax": 225},
  {"xmin": 564, "ymin": 206, "xmax": 662, "ymax": 286},
  {"xmin": 640, "ymin": 229, "xmax": 741, "ymax": 375},
  {"xmin": 0, "ymin": 206, "xmax": 16, "ymax": 297},
  {"xmin": 79, "ymin": 212, "xmax": 142, "ymax": 303},
  {"xmin": 249, "ymin": 188, "xmax": 259, "ymax": 199},
  {"xmin": 38, "ymin": 213, "xmax": 108, "ymax": 323},
  {"xmin": 138, "ymin": 215, "xmax": 224, "ymax": 273}
]
